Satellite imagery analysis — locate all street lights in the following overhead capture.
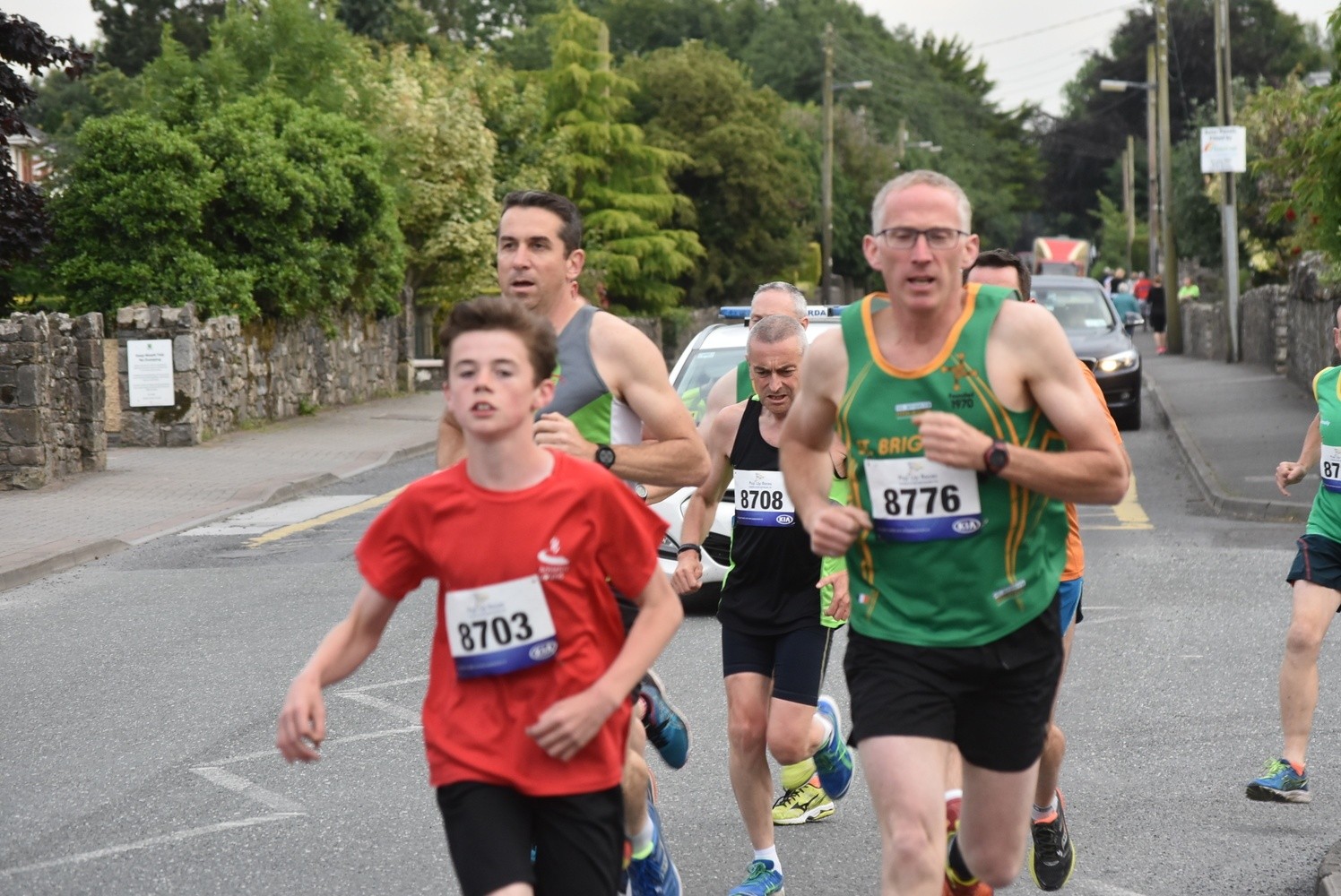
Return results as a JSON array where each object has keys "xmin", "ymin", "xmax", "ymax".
[
  {"xmin": 821, "ymin": 47, "xmax": 874, "ymax": 303},
  {"xmin": 1099, "ymin": 77, "xmax": 1186, "ymax": 355}
]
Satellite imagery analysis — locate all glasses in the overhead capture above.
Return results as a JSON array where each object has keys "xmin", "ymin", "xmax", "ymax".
[{"xmin": 874, "ymin": 227, "xmax": 969, "ymax": 250}]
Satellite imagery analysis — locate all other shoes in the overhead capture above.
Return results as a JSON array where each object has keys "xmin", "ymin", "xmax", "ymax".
[
  {"xmin": 1156, "ymin": 346, "xmax": 1169, "ymax": 355},
  {"xmin": 647, "ymin": 769, "xmax": 657, "ymax": 805}
]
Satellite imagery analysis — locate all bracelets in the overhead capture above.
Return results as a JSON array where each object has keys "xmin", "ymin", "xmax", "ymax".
[{"xmin": 677, "ymin": 543, "xmax": 702, "ymax": 561}]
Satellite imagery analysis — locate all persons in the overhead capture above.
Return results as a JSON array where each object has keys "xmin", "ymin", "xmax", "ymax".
[
  {"xmin": 1102, "ymin": 267, "xmax": 1199, "ymax": 353},
  {"xmin": 436, "ymin": 170, "xmax": 1132, "ymax": 896},
  {"xmin": 273, "ymin": 295, "xmax": 683, "ymax": 896},
  {"xmin": 1245, "ymin": 305, "xmax": 1341, "ymax": 803}
]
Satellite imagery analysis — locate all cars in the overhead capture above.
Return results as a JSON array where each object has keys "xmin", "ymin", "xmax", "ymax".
[
  {"xmin": 629, "ymin": 304, "xmax": 852, "ymax": 618},
  {"xmin": 1029, "ymin": 275, "xmax": 1147, "ymax": 431}
]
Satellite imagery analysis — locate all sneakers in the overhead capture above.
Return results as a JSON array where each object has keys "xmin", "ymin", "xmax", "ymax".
[
  {"xmin": 1029, "ymin": 787, "xmax": 1075, "ymax": 891},
  {"xmin": 640, "ymin": 669, "xmax": 692, "ymax": 769},
  {"xmin": 629, "ymin": 799, "xmax": 683, "ymax": 896},
  {"xmin": 772, "ymin": 783, "xmax": 835, "ymax": 825},
  {"xmin": 944, "ymin": 789, "xmax": 995, "ymax": 896},
  {"xmin": 780, "ymin": 756, "xmax": 818, "ymax": 790},
  {"xmin": 813, "ymin": 694, "xmax": 854, "ymax": 800},
  {"xmin": 729, "ymin": 859, "xmax": 785, "ymax": 896},
  {"xmin": 1245, "ymin": 757, "xmax": 1314, "ymax": 803}
]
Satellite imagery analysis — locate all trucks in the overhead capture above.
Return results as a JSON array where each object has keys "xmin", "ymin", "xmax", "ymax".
[{"xmin": 1032, "ymin": 235, "xmax": 1095, "ymax": 277}]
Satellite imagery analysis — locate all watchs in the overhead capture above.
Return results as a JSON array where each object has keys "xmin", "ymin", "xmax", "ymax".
[
  {"xmin": 595, "ymin": 443, "xmax": 616, "ymax": 470},
  {"xmin": 633, "ymin": 482, "xmax": 649, "ymax": 501},
  {"xmin": 982, "ymin": 439, "xmax": 1009, "ymax": 478}
]
